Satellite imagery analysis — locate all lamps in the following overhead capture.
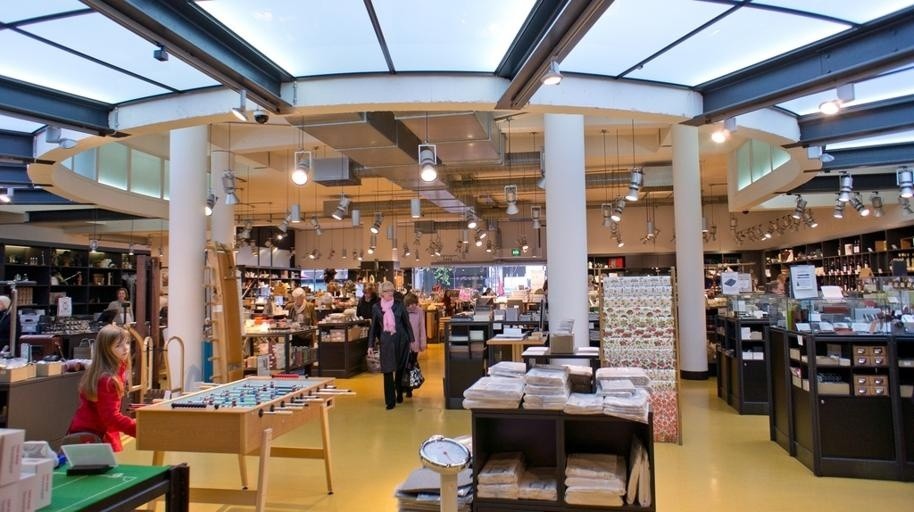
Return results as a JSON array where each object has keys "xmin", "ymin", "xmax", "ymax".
[
  {"xmin": 290, "ymin": 116, "xmax": 321, "ymax": 185},
  {"xmin": 216, "ymin": 184, "xmax": 497, "ymax": 261},
  {"xmin": 700, "ymin": 82, "xmax": 914, "ymax": 245},
  {"xmin": 501, "ymin": 113, "xmax": 546, "ymax": 252},
  {"xmin": 0, "ymin": 109, "xmax": 165, "ymax": 258},
  {"xmin": 412, "ymin": 112, "xmax": 442, "ymax": 185},
  {"xmin": 593, "ymin": 118, "xmax": 664, "ymax": 249},
  {"xmin": 153, "ymin": 41, "xmax": 169, "ymax": 62},
  {"xmin": 202, "ymin": 122, "xmax": 244, "ymax": 217}
]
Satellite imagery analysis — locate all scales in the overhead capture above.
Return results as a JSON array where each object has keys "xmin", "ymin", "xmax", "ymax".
[{"xmin": 419, "ymin": 434, "xmax": 470, "ymax": 512}]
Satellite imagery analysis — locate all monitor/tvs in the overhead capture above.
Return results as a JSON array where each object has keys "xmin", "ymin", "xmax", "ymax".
[
  {"xmin": 300, "ymin": 269, "xmax": 348, "ymax": 292},
  {"xmin": 274, "ymin": 296, "xmax": 283, "ymax": 306},
  {"xmin": 96, "ymin": 310, "xmax": 118, "ymax": 322},
  {"xmin": 355, "ymin": 283, "xmax": 364, "ymax": 297},
  {"xmin": 891, "ymin": 261, "xmax": 907, "ymax": 276}
]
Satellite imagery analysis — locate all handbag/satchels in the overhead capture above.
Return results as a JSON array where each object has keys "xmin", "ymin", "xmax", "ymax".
[{"xmin": 401, "ymin": 354, "xmax": 425, "ymax": 389}]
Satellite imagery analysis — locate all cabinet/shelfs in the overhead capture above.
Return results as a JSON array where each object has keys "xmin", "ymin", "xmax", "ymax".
[
  {"xmin": 0, "ymin": 244, "xmax": 159, "ymax": 442},
  {"xmin": 439, "ymin": 262, "xmax": 684, "ymax": 511},
  {"xmin": 236, "ymin": 264, "xmax": 439, "ymax": 377},
  {"xmin": 704, "ymin": 225, "xmax": 914, "ymax": 480}
]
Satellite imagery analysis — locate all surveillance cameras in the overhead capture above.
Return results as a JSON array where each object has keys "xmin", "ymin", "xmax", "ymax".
[
  {"xmin": 254, "ymin": 111, "xmax": 269, "ymax": 124},
  {"xmin": 742, "ymin": 211, "xmax": 749, "ymax": 214}
]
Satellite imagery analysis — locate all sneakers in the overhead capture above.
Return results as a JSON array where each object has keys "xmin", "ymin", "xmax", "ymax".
[{"xmin": 385, "ymin": 391, "xmax": 413, "ymax": 410}]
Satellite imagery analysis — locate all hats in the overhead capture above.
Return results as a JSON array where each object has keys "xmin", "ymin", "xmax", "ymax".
[{"xmin": 291, "ymin": 287, "xmax": 306, "ymax": 297}]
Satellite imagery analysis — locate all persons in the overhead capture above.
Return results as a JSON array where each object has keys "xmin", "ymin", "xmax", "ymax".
[
  {"xmin": 64, "ymin": 324, "xmax": 136, "ymax": 452},
  {"xmin": 288, "ymin": 287, "xmax": 318, "ymax": 376},
  {"xmin": 357, "ymin": 280, "xmax": 427, "ymax": 409},
  {"xmin": 106, "ymin": 288, "xmax": 134, "ymax": 325}
]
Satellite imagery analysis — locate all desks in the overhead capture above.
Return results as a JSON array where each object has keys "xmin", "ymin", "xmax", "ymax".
[{"xmin": 27, "ymin": 450, "xmax": 190, "ymax": 511}]
